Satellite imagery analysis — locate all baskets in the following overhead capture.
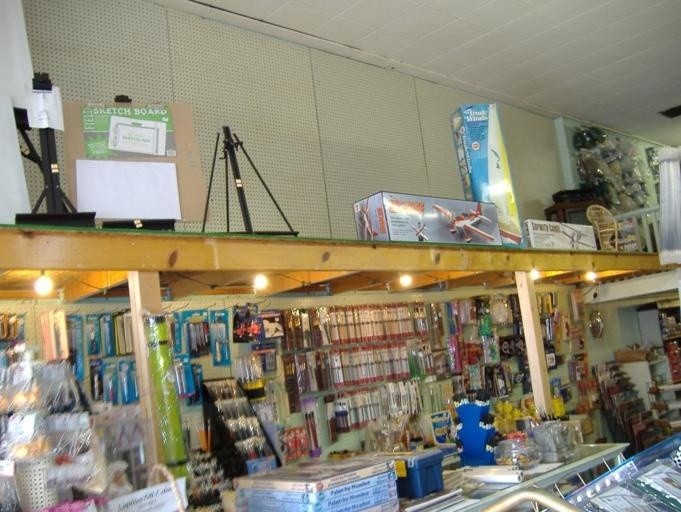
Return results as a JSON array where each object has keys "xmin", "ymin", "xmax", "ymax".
[{"xmin": 14, "ymin": 459, "xmax": 59, "ymax": 511}]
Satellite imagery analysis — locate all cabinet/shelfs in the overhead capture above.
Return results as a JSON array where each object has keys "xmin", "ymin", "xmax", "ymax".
[
  {"xmin": 618, "ymin": 355, "xmax": 681, "ymax": 421},
  {"xmin": 442, "ymin": 442, "xmax": 629, "ymax": 512}
]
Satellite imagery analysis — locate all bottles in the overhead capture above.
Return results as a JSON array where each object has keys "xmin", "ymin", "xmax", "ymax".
[{"xmin": 409, "ymin": 432, "xmax": 423, "ymax": 450}]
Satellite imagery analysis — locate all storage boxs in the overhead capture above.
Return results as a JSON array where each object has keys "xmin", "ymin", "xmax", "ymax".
[{"xmin": 344, "ymin": 449, "xmax": 443, "ymax": 498}]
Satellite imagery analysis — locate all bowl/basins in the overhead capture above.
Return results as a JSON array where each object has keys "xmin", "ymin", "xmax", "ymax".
[{"xmin": 493, "ymin": 438, "xmax": 543, "ymax": 469}]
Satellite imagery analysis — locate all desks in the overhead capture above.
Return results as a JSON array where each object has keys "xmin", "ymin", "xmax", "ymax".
[{"xmin": 544, "ymin": 200, "xmax": 604, "ymax": 223}]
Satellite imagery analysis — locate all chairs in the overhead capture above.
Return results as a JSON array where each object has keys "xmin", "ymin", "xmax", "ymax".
[{"xmin": 586, "ymin": 204, "xmax": 642, "ymax": 252}]
